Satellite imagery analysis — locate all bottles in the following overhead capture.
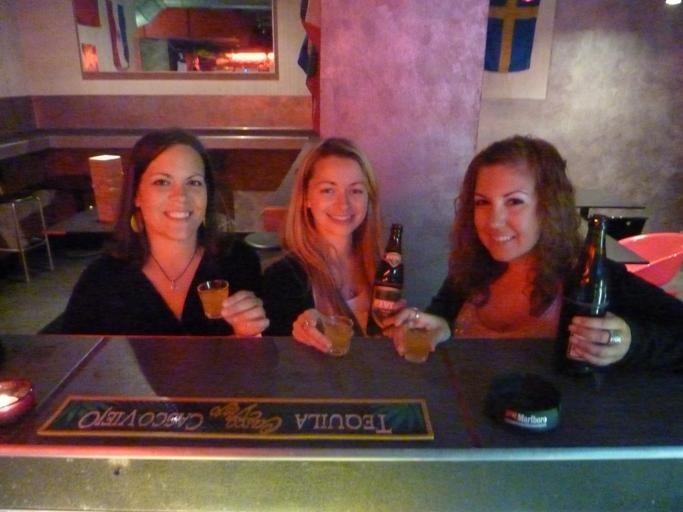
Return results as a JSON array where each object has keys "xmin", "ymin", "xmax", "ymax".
[
  {"xmin": 367, "ymin": 223, "xmax": 405, "ymax": 340},
  {"xmin": 554, "ymin": 214, "xmax": 610, "ymax": 371}
]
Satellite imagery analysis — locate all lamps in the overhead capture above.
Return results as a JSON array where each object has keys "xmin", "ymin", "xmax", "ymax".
[{"xmin": 88, "ymin": 154, "xmax": 124, "ymax": 225}]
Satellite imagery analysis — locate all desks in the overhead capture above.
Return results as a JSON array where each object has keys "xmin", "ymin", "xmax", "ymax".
[
  {"xmin": 48, "ymin": 208, "xmax": 133, "ymax": 266},
  {"xmin": 0, "ymin": 330, "xmax": 683, "ymax": 510}
]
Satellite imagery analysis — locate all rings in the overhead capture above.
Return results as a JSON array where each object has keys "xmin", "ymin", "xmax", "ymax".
[
  {"xmin": 303, "ymin": 320, "xmax": 316, "ymax": 329},
  {"xmin": 415, "ymin": 311, "xmax": 420, "ymax": 322},
  {"xmin": 605, "ymin": 330, "xmax": 623, "ymax": 346}
]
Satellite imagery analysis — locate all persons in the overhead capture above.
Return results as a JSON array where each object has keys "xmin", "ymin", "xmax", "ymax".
[
  {"xmin": 264, "ymin": 137, "xmax": 407, "ymax": 354},
  {"xmin": 49, "ymin": 129, "xmax": 270, "ymax": 338},
  {"xmin": 394, "ymin": 136, "xmax": 631, "ymax": 366}
]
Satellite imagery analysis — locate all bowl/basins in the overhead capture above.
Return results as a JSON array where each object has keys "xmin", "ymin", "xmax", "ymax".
[{"xmin": 0, "ymin": 380, "xmax": 36, "ymax": 426}]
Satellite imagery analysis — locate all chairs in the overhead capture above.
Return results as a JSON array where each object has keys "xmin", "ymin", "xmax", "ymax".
[{"xmin": 254, "ymin": 205, "xmax": 291, "ymax": 236}]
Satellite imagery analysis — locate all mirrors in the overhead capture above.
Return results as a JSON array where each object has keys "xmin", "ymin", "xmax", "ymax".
[{"xmin": 71, "ymin": 0, "xmax": 279, "ymax": 82}]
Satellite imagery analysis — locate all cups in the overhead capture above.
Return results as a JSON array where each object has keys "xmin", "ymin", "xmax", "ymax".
[
  {"xmin": 401, "ymin": 306, "xmax": 436, "ymax": 363},
  {"xmin": 196, "ymin": 279, "xmax": 229, "ymax": 320},
  {"xmin": 321, "ymin": 314, "xmax": 355, "ymax": 359}
]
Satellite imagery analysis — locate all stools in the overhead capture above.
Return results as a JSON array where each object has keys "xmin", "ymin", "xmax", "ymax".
[
  {"xmin": 242, "ymin": 233, "xmax": 284, "ymax": 258},
  {"xmin": 0, "ymin": 191, "xmax": 55, "ymax": 283}
]
